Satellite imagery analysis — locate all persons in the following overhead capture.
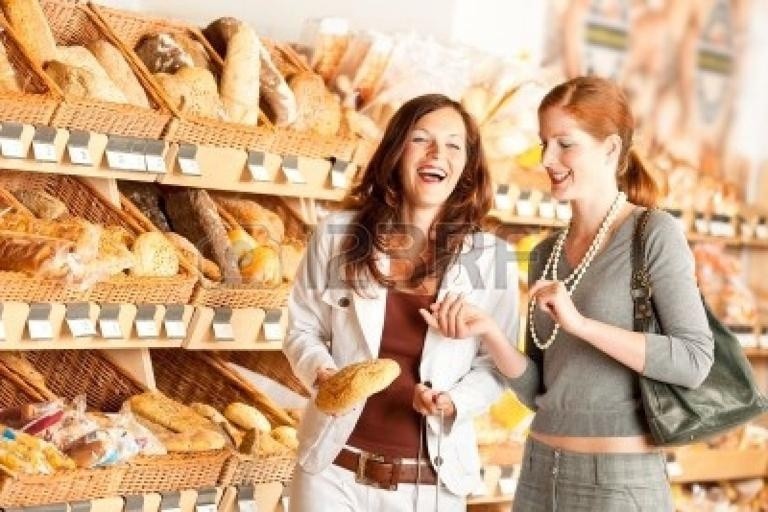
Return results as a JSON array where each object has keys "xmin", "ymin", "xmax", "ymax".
[
  {"xmin": 418, "ymin": 74, "xmax": 716, "ymax": 512},
  {"xmin": 278, "ymin": 92, "xmax": 523, "ymax": 511}
]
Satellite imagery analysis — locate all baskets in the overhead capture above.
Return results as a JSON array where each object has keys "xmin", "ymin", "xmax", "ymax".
[
  {"xmin": 0, "ymin": 3, "xmax": 384, "ymax": 170},
  {"xmin": 1, "ymin": 172, "xmax": 318, "ymax": 312},
  {"xmin": 0, "ymin": 348, "xmax": 312, "ymax": 508}
]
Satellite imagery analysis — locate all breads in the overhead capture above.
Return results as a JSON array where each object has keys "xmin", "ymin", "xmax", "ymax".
[
  {"xmin": 87, "ymin": 392, "xmax": 300, "ymax": 459},
  {"xmin": 1, "ymin": 190, "xmax": 176, "ymax": 291},
  {"xmin": 1, "ymin": 351, "xmax": 46, "ymax": 388},
  {"xmin": 136, "ymin": 16, "xmax": 342, "ymax": 140},
  {"xmin": 315, "ymin": 29, "xmax": 386, "ymax": 138},
  {"xmin": 315, "ymin": 357, "xmax": 401, "ymax": 416},
  {"xmin": 1, "ymin": 405, "xmax": 105, "ymax": 470},
  {"xmin": 0, "ymin": 0, "xmax": 152, "ymax": 108},
  {"xmin": 118, "ymin": 179, "xmax": 308, "ymax": 287}
]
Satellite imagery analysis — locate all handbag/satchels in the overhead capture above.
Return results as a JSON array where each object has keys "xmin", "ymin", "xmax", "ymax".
[{"xmin": 632, "ymin": 289, "xmax": 768, "ymax": 446}]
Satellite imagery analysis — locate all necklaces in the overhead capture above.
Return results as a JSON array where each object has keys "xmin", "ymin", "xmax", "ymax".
[{"xmin": 531, "ymin": 192, "xmax": 637, "ymax": 350}]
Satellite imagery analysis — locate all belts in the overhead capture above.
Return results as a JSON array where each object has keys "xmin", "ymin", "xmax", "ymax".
[{"xmin": 331, "ymin": 448, "xmax": 437, "ymax": 491}]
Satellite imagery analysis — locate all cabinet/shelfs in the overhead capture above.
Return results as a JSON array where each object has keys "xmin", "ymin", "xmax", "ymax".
[
  {"xmin": 483, "ymin": 207, "xmax": 768, "ymax": 512},
  {"xmin": 0, "ymin": 120, "xmax": 357, "ymax": 512}
]
